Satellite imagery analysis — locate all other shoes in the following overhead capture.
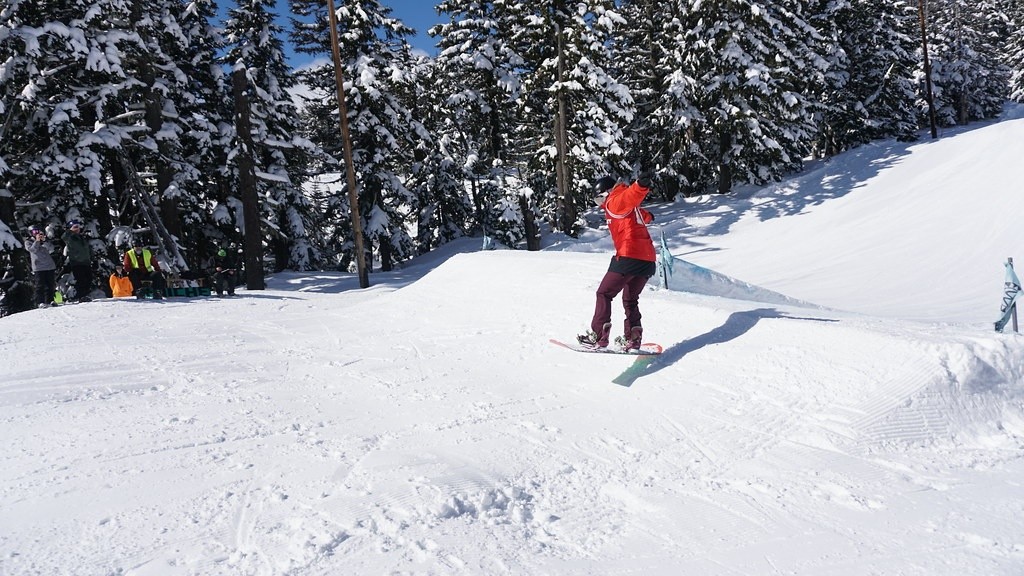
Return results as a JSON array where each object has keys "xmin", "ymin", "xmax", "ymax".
[
  {"xmin": 576, "ymin": 333, "xmax": 606, "ymax": 349},
  {"xmin": 135, "ymin": 287, "xmax": 146, "ymax": 300},
  {"xmin": 38, "ymin": 299, "xmax": 59, "ymax": 308},
  {"xmin": 614, "ymin": 335, "xmax": 641, "ymax": 349},
  {"xmin": 228, "ymin": 290, "xmax": 236, "ymax": 297},
  {"xmin": 217, "ymin": 291, "xmax": 224, "ymax": 298},
  {"xmin": 153, "ymin": 288, "xmax": 163, "ymax": 300},
  {"xmin": 78, "ymin": 293, "xmax": 92, "ymax": 304}
]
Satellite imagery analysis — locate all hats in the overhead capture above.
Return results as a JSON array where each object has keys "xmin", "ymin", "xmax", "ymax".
[{"xmin": 218, "ymin": 249, "xmax": 228, "ymax": 256}]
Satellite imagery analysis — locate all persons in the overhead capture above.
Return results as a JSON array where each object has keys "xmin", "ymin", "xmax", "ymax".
[
  {"xmin": 61, "ymin": 220, "xmax": 94, "ymax": 302},
  {"xmin": 109, "ymin": 238, "xmax": 165, "ymax": 299},
  {"xmin": 576, "ymin": 172, "xmax": 656, "ymax": 353},
  {"xmin": 25, "ymin": 225, "xmax": 58, "ymax": 308},
  {"xmin": 205, "ymin": 250, "xmax": 237, "ymax": 298}
]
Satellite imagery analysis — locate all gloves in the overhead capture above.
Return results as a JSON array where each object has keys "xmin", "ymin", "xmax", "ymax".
[
  {"xmin": 648, "ymin": 211, "xmax": 655, "ymax": 224},
  {"xmin": 637, "ymin": 170, "xmax": 653, "ymax": 187}
]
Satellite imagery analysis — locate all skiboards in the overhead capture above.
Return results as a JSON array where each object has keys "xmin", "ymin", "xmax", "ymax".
[{"xmin": 541, "ymin": 324, "xmax": 677, "ymax": 364}]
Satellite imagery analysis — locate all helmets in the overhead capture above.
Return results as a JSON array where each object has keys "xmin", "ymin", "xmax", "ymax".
[
  {"xmin": 27, "ymin": 224, "xmax": 40, "ymax": 236},
  {"xmin": 130, "ymin": 239, "xmax": 143, "ymax": 247},
  {"xmin": 66, "ymin": 219, "xmax": 82, "ymax": 230},
  {"xmin": 591, "ymin": 176, "xmax": 616, "ymax": 199}
]
